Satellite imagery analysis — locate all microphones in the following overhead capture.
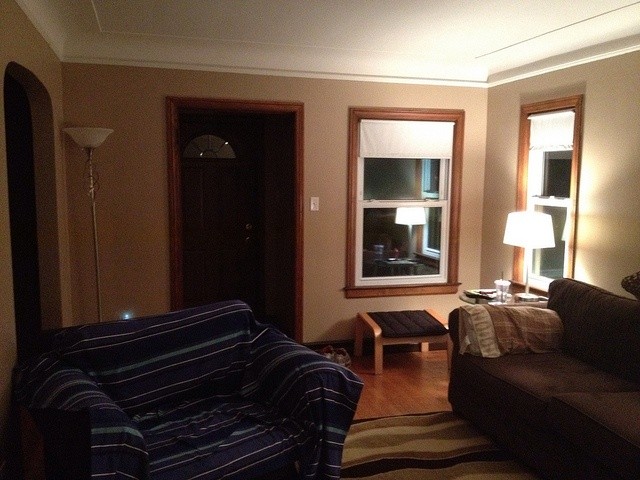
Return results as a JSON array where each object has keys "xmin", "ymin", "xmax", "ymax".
[{"xmin": 354, "ymin": 309, "xmax": 453, "ymax": 377}]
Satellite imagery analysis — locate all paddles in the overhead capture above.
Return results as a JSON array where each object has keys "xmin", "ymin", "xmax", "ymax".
[{"xmin": 341, "ymin": 411, "xmax": 546, "ymax": 479}]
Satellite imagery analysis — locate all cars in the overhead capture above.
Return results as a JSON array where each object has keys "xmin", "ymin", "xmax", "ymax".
[
  {"xmin": 374, "ymin": 245, "xmax": 383, "ymax": 261},
  {"xmin": 494, "ymin": 280, "xmax": 511, "ymax": 303}
]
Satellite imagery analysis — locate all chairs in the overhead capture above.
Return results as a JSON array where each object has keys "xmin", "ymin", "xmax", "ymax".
[{"xmin": 314, "ymin": 345, "xmax": 352, "ymax": 368}]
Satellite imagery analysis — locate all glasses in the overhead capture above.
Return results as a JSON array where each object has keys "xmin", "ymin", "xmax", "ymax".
[
  {"xmin": 63, "ymin": 126, "xmax": 114, "ymax": 324},
  {"xmin": 395, "ymin": 201, "xmax": 427, "ymax": 262},
  {"xmin": 503, "ymin": 210, "xmax": 556, "ymax": 303}
]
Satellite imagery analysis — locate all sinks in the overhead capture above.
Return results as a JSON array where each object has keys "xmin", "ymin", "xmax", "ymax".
[
  {"xmin": 448, "ymin": 278, "xmax": 639, "ymax": 479},
  {"xmin": 46, "ymin": 299, "xmax": 346, "ymax": 480},
  {"xmin": 364, "ymin": 251, "xmax": 388, "ymax": 278}
]
